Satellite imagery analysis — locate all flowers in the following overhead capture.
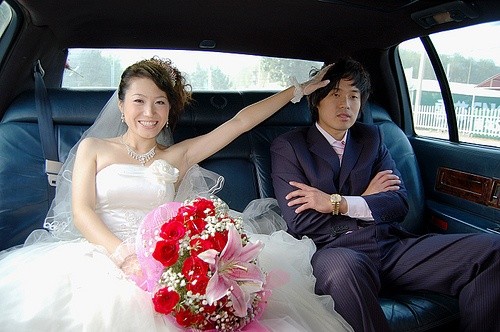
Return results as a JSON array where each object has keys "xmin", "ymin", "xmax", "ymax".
[{"xmin": 120, "ymin": 197, "xmax": 291, "ymax": 332}]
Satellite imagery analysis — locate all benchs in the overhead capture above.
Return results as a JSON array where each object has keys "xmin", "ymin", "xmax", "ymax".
[{"xmin": 0, "ymin": 89, "xmax": 440, "ymax": 332}]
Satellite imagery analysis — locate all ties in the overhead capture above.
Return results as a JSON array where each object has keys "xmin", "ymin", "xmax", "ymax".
[{"xmin": 332, "ymin": 141, "xmax": 345, "ymax": 165}]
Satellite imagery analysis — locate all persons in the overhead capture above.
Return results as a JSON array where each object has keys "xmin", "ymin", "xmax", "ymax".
[
  {"xmin": 269, "ymin": 56, "xmax": 499, "ymax": 332},
  {"xmin": 0, "ymin": 57, "xmax": 336, "ymax": 332}
]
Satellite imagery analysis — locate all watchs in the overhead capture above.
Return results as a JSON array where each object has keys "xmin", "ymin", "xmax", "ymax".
[{"xmin": 329, "ymin": 194, "xmax": 341, "ymax": 215}]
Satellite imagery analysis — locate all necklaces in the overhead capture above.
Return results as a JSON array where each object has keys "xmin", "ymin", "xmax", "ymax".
[{"xmin": 120, "ymin": 134, "xmax": 158, "ymax": 167}]
